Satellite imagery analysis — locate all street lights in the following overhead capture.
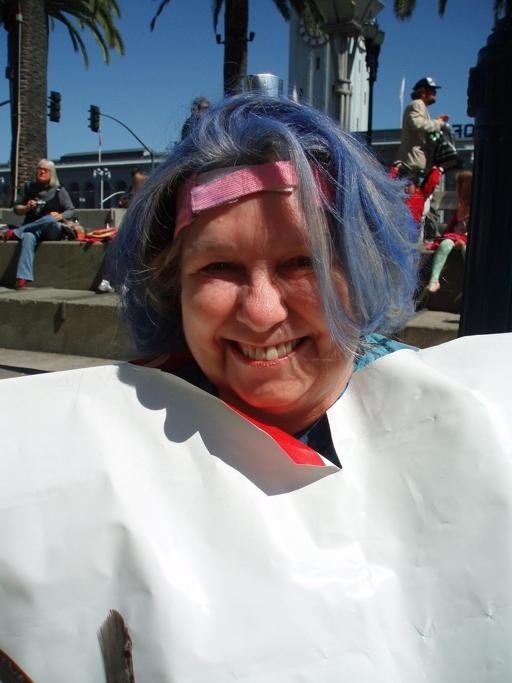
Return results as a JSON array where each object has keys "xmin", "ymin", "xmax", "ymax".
[{"xmin": 92, "ymin": 166, "xmax": 111, "ymax": 209}]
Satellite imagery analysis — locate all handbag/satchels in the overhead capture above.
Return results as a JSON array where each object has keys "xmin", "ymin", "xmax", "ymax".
[{"xmin": 425, "ymin": 123, "xmax": 462, "ymax": 174}]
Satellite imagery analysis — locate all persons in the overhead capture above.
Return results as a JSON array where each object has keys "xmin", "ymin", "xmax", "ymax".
[
  {"xmin": 98, "ymin": 245, "xmax": 151, "ymax": 296},
  {"xmin": 0, "ymin": 89, "xmax": 511, "ymax": 682},
  {"xmin": 426, "ymin": 171, "xmax": 473, "ymax": 292},
  {"xmin": 2, "ymin": 158, "xmax": 78, "ymax": 290},
  {"xmin": 181, "ymin": 94, "xmax": 211, "ymax": 141},
  {"xmin": 130, "ymin": 164, "xmax": 148, "ymax": 192},
  {"xmin": 394, "ymin": 74, "xmax": 450, "ymax": 242},
  {"xmin": 455, "ymin": 11, "xmax": 511, "ymax": 338},
  {"xmin": 388, "ymin": 160, "xmax": 444, "ymax": 239}
]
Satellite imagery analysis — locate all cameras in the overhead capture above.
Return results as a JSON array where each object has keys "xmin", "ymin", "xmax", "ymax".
[{"xmin": 34, "ymin": 200, "xmax": 46, "ymax": 207}]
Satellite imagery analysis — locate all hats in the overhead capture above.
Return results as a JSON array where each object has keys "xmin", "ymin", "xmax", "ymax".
[{"xmin": 412, "ymin": 77, "xmax": 441, "ymax": 89}]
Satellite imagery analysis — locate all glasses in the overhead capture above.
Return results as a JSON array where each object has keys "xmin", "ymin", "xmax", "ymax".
[{"xmin": 36, "ymin": 166, "xmax": 55, "ymax": 174}]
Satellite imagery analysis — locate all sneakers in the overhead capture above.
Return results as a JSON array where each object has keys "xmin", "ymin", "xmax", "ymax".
[
  {"xmin": 17, "ymin": 279, "xmax": 27, "ymax": 288},
  {"xmin": 5, "ymin": 229, "xmax": 13, "ymax": 241},
  {"xmin": 425, "ymin": 283, "xmax": 440, "ymax": 293}
]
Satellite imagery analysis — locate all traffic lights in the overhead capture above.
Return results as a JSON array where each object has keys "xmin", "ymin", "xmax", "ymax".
[
  {"xmin": 48, "ymin": 90, "xmax": 62, "ymax": 123},
  {"xmin": 88, "ymin": 103, "xmax": 101, "ymax": 134}
]
